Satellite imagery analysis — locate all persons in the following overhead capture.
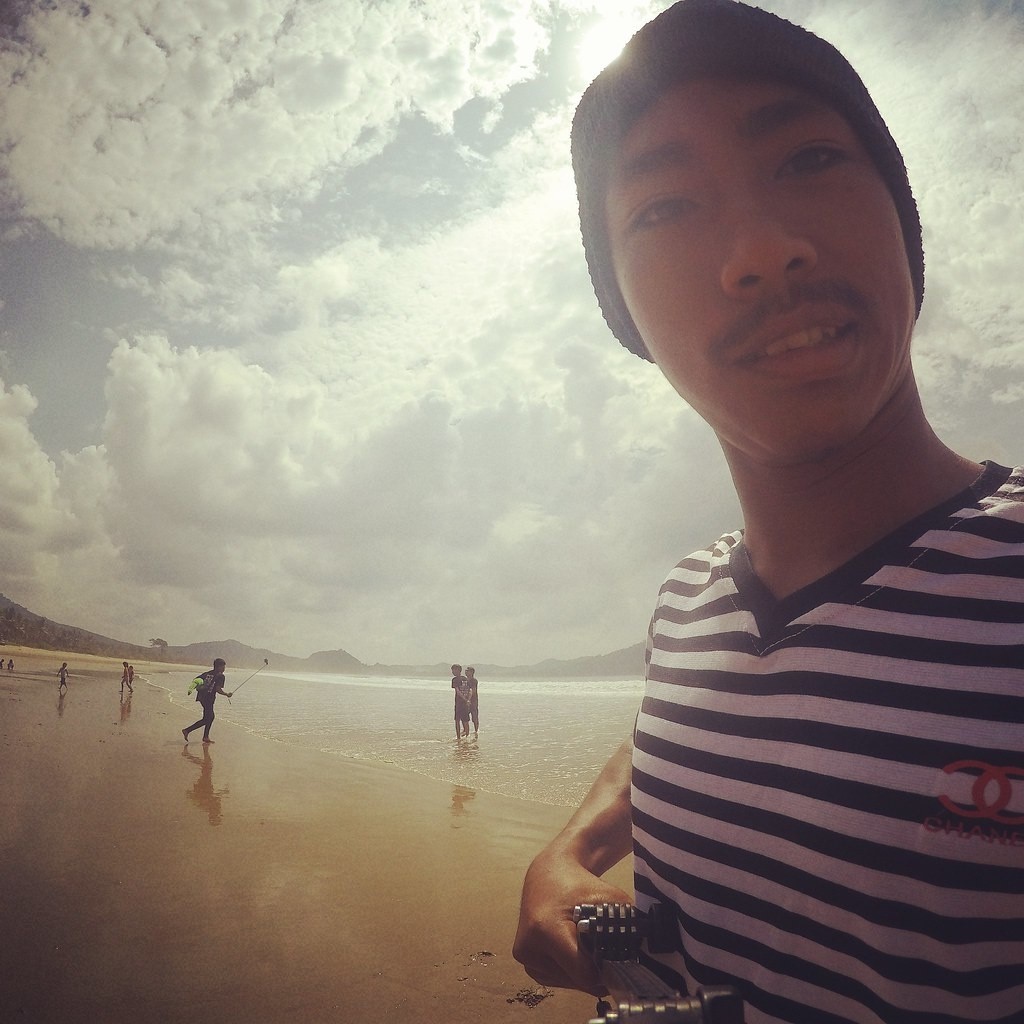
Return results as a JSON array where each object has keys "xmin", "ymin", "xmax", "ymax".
[
  {"xmin": 118, "ymin": 662, "xmax": 135, "ymax": 693},
  {"xmin": 7, "ymin": 659, "xmax": 14, "ymax": 670},
  {"xmin": 511, "ymin": 0, "xmax": 1024, "ymax": 1024},
  {"xmin": 57, "ymin": 663, "xmax": 69, "ymax": 689},
  {"xmin": 450, "ymin": 664, "xmax": 479, "ymax": 738},
  {"xmin": 0, "ymin": 659, "xmax": 4, "ymax": 669},
  {"xmin": 182, "ymin": 657, "xmax": 232, "ymax": 742}
]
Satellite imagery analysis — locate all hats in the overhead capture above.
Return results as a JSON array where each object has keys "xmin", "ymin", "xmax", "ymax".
[{"xmin": 569, "ymin": 0, "xmax": 926, "ymax": 365}]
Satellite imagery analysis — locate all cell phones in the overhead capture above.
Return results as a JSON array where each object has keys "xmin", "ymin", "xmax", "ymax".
[{"xmin": 264, "ymin": 659, "xmax": 268, "ymax": 662}]
